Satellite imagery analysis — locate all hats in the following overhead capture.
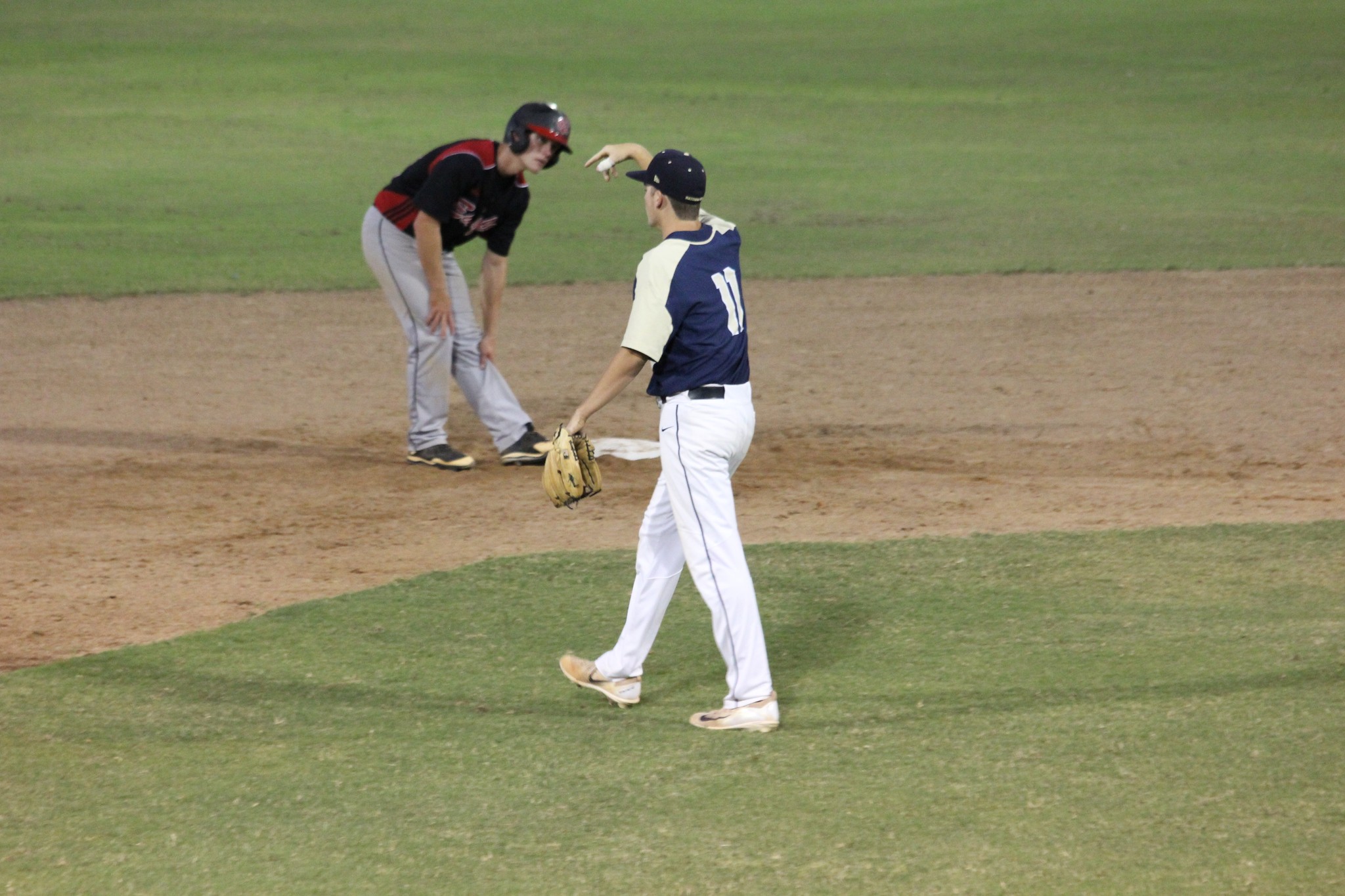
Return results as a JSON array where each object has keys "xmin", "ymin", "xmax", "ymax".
[{"xmin": 626, "ymin": 149, "xmax": 707, "ymax": 205}]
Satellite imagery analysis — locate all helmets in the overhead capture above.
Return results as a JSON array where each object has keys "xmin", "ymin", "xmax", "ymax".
[{"xmin": 509, "ymin": 102, "xmax": 574, "ymax": 168}]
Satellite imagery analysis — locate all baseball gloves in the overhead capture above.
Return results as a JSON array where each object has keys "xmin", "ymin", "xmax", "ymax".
[{"xmin": 540, "ymin": 425, "xmax": 602, "ymax": 508}]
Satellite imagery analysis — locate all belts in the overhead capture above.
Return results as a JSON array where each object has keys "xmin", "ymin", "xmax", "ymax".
[{"xmin": 660, "ymin": 385, "xmax": 725, "ymax": 404}]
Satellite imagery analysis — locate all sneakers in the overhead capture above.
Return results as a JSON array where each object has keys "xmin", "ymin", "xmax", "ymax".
[
  {"xmin": 560, "ymin": 654, "xmax": 642, "ymax": 709},
  {"xmin": 500, "ymin": 430, "xmax": 553, "ymax": 467},
  {"xmin": 406, "ymin": 445, "xmax": 476, "ymax": 473},
  {"xmin": 690, "ymin": 690, "xmax": 779, "ymax": 733}
]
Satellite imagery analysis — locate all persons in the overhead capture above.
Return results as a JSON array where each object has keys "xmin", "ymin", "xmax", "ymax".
[
  {"xmin": 360, "ymin": 100, "xmax": 575, "ymax": 471},
  {"xmin": 557, "ymin": 141, "xmax": 780, "ymax": 729}
]
